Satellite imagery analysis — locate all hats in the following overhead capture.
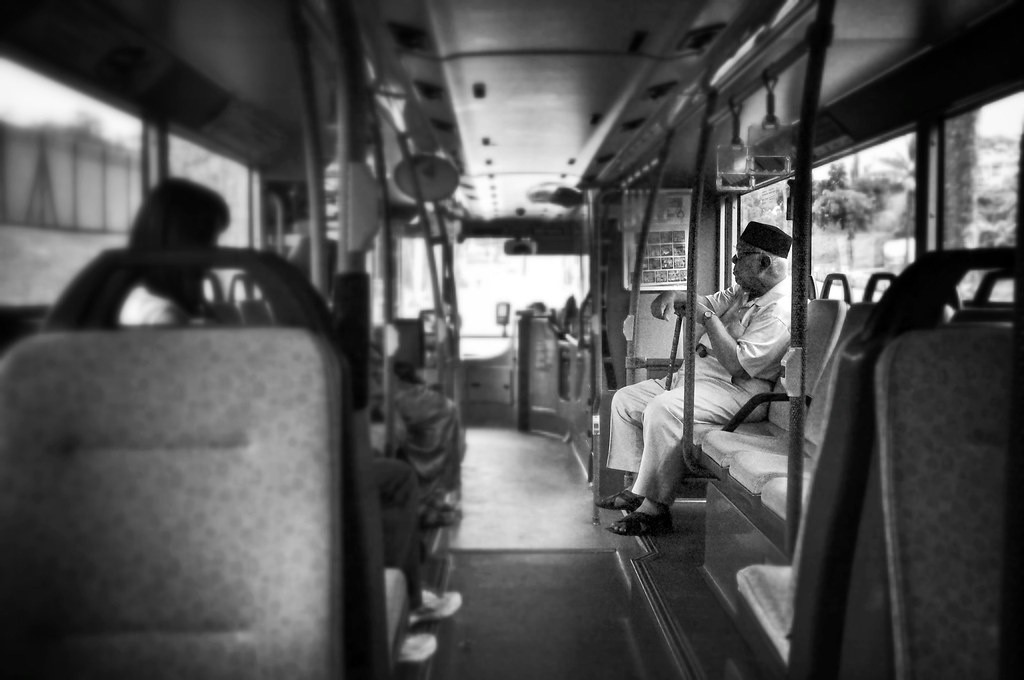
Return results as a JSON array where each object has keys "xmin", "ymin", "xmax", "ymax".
[{"xmin": 739, "ymin": 221, "xmax": 793, "ymax": 258}]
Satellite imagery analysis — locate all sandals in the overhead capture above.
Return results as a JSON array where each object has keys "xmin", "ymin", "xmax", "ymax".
[
  {"xmin": 595, "ymin": 489, "xmax": 647, "ymax": 511},
  {"xmin": 606, "ymin": 512, "xmax": 674, "ymax": 537}
]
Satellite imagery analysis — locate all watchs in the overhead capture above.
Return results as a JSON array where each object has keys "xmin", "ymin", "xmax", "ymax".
[{"xmin": 702, "ymin": 310, "xmax": 715, "ymax": 326}]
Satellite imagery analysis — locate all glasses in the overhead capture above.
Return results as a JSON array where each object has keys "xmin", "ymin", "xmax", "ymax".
[{"xmin": 734, "ymin": 247, "xmax": 762, "ymax": 261}]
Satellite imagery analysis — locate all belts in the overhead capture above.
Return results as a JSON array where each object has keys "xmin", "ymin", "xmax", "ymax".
[{"xmin": 696, "ymin": 343, "xmax": 714, "ymax": 358}]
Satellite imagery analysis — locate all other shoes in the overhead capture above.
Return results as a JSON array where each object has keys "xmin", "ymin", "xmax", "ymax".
[
  {"xmin": 410, "ymin": 587, "xmax": 469, "ymax": 626},
  {"xmin": 399, "ymin": 633, "xmax": 446, "ymax": 661}
]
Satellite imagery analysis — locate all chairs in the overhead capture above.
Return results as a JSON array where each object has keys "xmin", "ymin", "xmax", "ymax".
[
  {"xmin": 0, "ymin": 245, "xmax": 412, "ymax": 680},
  {"xmin": 678, "ymin": 246, "xmax": 1019, "ymax": 680}
]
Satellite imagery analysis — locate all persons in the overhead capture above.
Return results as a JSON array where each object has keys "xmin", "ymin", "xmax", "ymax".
[
  {"xmin": 594, "ymin": 221, "xmax": 791, "ymax": 536},
  {"xmin": 116, "ymin": 177, "xmax": 464, "ymax": 660}
]
